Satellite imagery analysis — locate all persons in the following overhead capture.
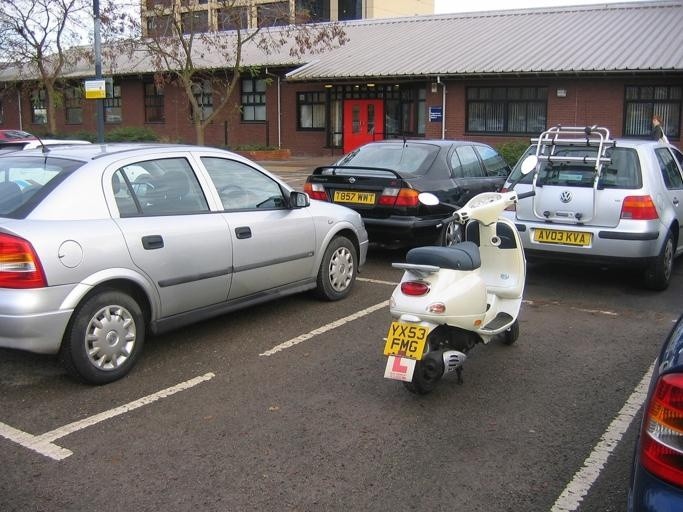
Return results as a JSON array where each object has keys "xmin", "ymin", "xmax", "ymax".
[{"xmin": 647, "ymin": 114, "xmax": 669, "ymax": 144}]
